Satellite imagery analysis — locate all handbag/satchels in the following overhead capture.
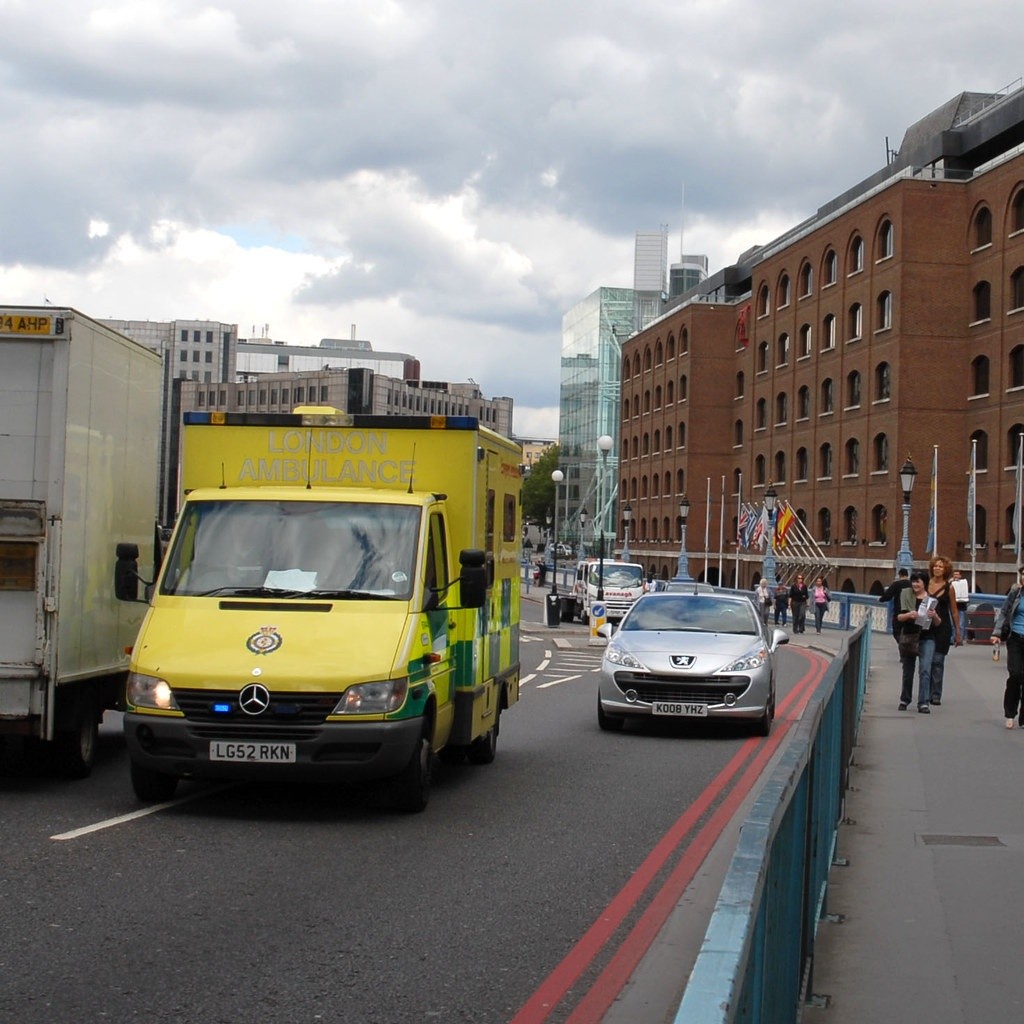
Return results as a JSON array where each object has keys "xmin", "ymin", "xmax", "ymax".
[
  {"xmin": 1001, "ymin": 620, "xmax": 1011, "ymax": 642},
  {"xmin": 764, "ymin": 597, "xmax": 772, "ymax": 606},
  {"xmin": 898, "ymin": 635, "xmax": 922, "ymax": 657}
]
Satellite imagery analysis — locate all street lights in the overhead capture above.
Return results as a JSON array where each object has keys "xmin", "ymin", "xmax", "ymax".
[
  {"xmin": 895, "ymin": 457, "xmax": 917, "ymax": 584},
  {"xmin": 578, "ymin": 509, "xmax": 587, "ymax": 561},
  {"xmin": 621, "ymin": 501, "xmax": 633, "ymax": 564},
  {"xmin": 764, "ymin": 484, "xmax": 778, "ymax": 590},
  {"xmin": 598, "ymin": 435, "xmax": 614, "ymax": 601},
  {"xmin": 673, "ymin": 494, "xmax": 694, "ymax": 581},
  {"xmin": 545, "ymin": 512, "xmax": 552, "ymax": 556},
  {"xmin": 551, "ymin": 470, "xmax": 564, "ymax": 592}
]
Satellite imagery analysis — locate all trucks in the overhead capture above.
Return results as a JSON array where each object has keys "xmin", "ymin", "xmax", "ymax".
[
  {"xmin": 574, "ymin": 560, "xmax": 644, "ymax": 622},
  {"xmin": 0, "ymin": 305, "xmax": 181, "ymax": 774}
]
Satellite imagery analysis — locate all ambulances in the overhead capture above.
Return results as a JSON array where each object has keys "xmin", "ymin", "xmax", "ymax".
[{"xmin": 113, "ymin": 411, "xmax": 523, "ymax": 800}]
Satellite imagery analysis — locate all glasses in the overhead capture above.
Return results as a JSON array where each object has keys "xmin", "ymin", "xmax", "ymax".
[{"xmin": 911, "ymin": 571, "xmax": 923, "ymax": 577}]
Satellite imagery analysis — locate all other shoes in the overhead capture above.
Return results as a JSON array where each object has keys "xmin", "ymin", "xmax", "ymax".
[
  {"xmin": 930, "ymin": 699, "xmax": 942, "ymax": 706},
  {"xmin": 1005, "ymin": 718, "xmax": 1014, "ymax": 729},
  {"xmin": 899, "ymin": 703, "xmax": 907, "ymax": 710},
  {"xmin": 918, "ymin": 704, "xmax": 931, "ymax": 714}
]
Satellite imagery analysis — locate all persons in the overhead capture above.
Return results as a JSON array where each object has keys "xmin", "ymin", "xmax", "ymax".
[
  {"xmin": 990, "ymin": 566, "xmax": 1024, "ymax": 728},
  {"xmin": 774, "ymin": 581, "xmax": 788, "ymax": 627},
  {"xmin": 790, "ymin": 575, "xmax": 809, "ymax": 633},
  {"xmin": 810, "ymin": 577, "xmax": 832, "ymax": 634},
  {"xmin": 536, "ymin": 560, "xmax": 546, "ymax": 584},
  {"xmin": 756, "ymin": 579, "xmax": 772, "ymax": 626},
  {"xmin": 927, "ymin": 556, "xmax": 969, "ymax": 705},
  {"xmin": 878, "ymin": 568, "xmax": 912, "ymax": 642},
  {"xmin": 897, "ymin": 572, "xmax": 942, "ymax": 714}
]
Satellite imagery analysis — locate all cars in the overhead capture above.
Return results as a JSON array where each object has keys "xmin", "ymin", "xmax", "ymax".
[
  {"xmin": 599, "ymin": 592, "xmax": 789, "ymax": 734},
  {"xmin": 646, "ymin": 580, "xmax": 716, "ymax": 612},
  {"xmin": 545, "ymin": 544, "xmax": 571, "ymax": 557}
]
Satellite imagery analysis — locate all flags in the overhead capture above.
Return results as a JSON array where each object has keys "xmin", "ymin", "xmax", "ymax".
[{"xmin": 738, "ymin": 506, "xmax": 796, "ymax": 551}]
